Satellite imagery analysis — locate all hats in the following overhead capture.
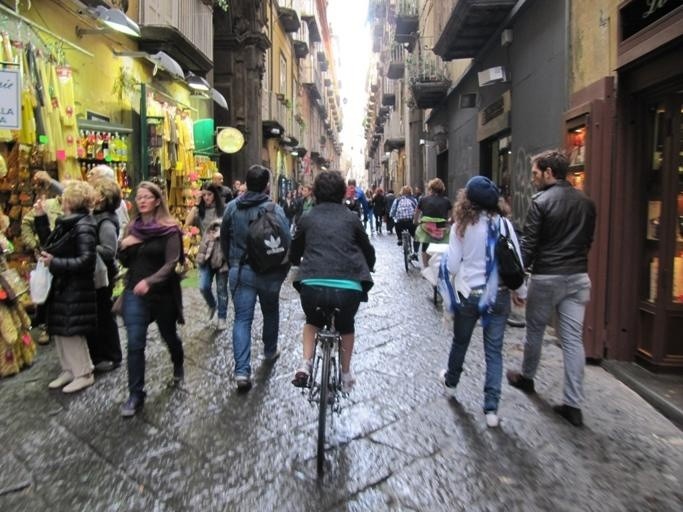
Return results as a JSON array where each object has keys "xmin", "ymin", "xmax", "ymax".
[{"xmin": 465, "ymin": 176, "xmax": 501, "ymax": 207}]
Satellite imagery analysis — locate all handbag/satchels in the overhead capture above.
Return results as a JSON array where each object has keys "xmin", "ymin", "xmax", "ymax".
[
  {"xmin": 494, "ymin": 233, "xmax": 525, "ymax": 290},
  {"xmin": 247, "ymin": 204, "xmax": 290, "ymax": 270}
]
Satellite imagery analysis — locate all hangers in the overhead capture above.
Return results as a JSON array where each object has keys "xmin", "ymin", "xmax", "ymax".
[{"xmin": 0, "ymin": 12, "xmax": 67, "ymax": 64}]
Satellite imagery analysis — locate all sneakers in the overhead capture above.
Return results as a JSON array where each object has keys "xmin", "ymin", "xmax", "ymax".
[
  {"xmin": 173, "ymin": 360, "xmax": 185, "ymax": 381},
  {"xmin": 264, "ymin": 348, "xmax": 280, "ymax": 360},
  {"xmin": 37, "ymin": 324, "xmax": 49, "ymax": 344},
  {"xmin": 485, "ymin": 411, "xmax": 502, "ymax": 429},
  {"xmin": 207, "ymin": 307, "xmax": 227, "ymax": 330},
  {"xmin": 439, "ymin": 367, "xmax": 457, "ymax": 396},
  {"xmin": 341, "ymin": 377, "xmax": 356, "ymax": 391},
  {"xmin": 236, "ymin": 376, "xmax": 251, "ymax": 391},
  {"xmin": 506, "ymin": 370, "xmax": 537, "ymax": 394},
  {"xmin": 116, "ymin": 393, "xmax": 146, "ymax": 418},
  {"xmin": 291, "ymin": 372, "xmax": 310, "ymax": 389},
  {"xmin": 45, "ymin": 359, "xmax": 120, "ymax": 393},
  {"xmin": 551, "ymin": 403, "xmax": 583, "ymax": 426},
  {"xmin": 410, "ymin": 253, "xmax": 419, "ymax": 261},
  {"xmin": 398, "ymin": 241, "xmax": 402, "ymax": 245}
]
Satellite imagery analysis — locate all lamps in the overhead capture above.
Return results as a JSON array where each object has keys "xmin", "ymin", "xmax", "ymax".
[
  {"xmin": 76, "ymin": 6, "xmax": 141, "ymax": 38},
  {"xmin": 112, "ymin": 51, "xmax": 228, "ymax": 110}
]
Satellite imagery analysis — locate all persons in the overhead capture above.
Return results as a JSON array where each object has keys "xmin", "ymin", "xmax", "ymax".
[
  {"xmin": 34, "ymin": 170, "xmax": 118, "ymax": 375},
  {"xmin": 185, "ymin": 181, "xmax": 229, "ymax": 330},
  {"xmin": 287, "ymin": 171, "xmax": 375, "ymax": 393},
  {"xmin": 345, "ymin": 175, "xmax": 513, "ymax": 281},
  {"xmin": 87, "ymin": 164, "xmax": 131, "ymax": 328},
  {"xmin": 116, "ymin": 180, "xmax": 185, "ymax": 418},
  {"xmin": 32, "ymin": 179, "xmax": 98, "ymax": 393},
  {"xmin": 231, "ymin": 176, "xmax": 315, "ymax": 239},
  {"xmin": 218, "ymin": 164, "xmax": 293, "ymax": 387},
  {"xmin": 437, "ymin": 174, "xmax": 524, "ymax": 429},
  {"xmin": 507, "ymin": 150, "xmax": 596, "ymax": 429},
  {"xmin": 199, "ymin": 172, "xmax": 231, "ymax": 204}
]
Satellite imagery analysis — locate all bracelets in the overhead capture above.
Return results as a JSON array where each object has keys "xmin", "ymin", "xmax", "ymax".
[{"xmin": 194, "ymin": 204, "xmax": 200, "ymax": 207}]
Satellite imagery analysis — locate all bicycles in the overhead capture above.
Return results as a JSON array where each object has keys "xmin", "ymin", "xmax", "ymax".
[
  {"xmin": 428, "ymin": 237, "xmax": 442, "ymax": 304},
  {"xmin": 504, "ymin": 228, "xmax": 533, "ymax": 328},
  {"xmin": 401, "ymin": 229, "xmax": 414, "ymax": 272},
  {"xmin": 306, "ymin": 297, "xmax": 347, "ymax": 474}
]
